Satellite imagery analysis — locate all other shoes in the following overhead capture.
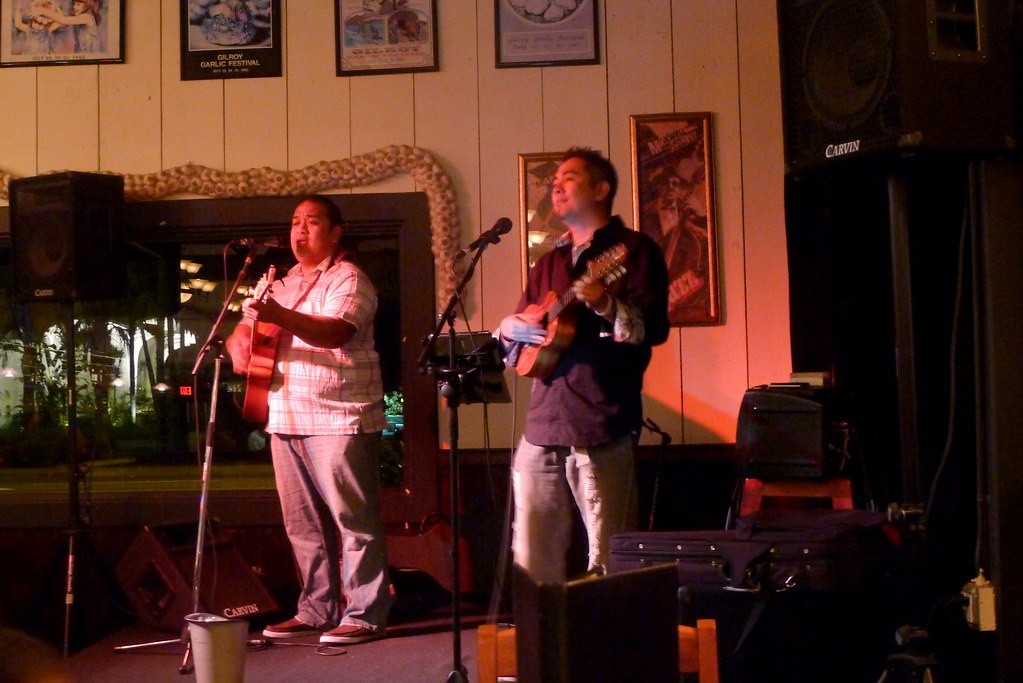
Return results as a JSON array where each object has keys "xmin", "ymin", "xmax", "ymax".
[
  {"xmin": 262, "ymin": 616, "xmax": 316, "ymax": 638},
  {"xmin": 321, "ymin": 624, "xmax": 379, "ymax": 645}
]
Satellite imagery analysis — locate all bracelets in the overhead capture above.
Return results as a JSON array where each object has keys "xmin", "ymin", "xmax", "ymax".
[{"xmin": 594, "ymin": 295, "xmax": 613, "ymax": 316}]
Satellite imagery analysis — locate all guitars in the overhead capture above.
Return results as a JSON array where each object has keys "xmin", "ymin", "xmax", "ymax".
[
  {"xmin": 240, "ymin": 263, "xmax": 285, "ymax": 427},
  {"xmin": 508, "ymin": 239, "xmax": 629, "ymax": 380}
]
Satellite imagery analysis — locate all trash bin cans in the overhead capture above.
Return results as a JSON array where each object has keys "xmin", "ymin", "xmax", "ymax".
[{"xmin": 185, "ymin": 613, "xmax": 249, "ymax": 683}]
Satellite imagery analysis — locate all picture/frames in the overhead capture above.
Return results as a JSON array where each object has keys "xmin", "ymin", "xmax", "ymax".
[
  {"xmin": 333, "ymin": 0, "xmax": 440, "ymax": 77},
  {"xmin": 179, "ymin": 0, "xmax": 282, "ymax": 81},
  {"xmin": 0, "ymin": 0, "xmax": 125, "ymax": 69},
  {"xmin": 494, "ymin": 0, "xmax": 600, "ymax": 69},
  {"xmin": 517, "ymin": 150, "xmax": 601, "ymax": 296},
  {"xmin": 629, "ymin": 112, "xmax": 721, "ymax": 327}
]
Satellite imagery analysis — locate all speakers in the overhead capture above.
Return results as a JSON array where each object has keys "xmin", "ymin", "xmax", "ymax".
[
  {"xmin": 8, "ymin": 171, "xmax": 125, "ymax": 303},
  {"xmin": 735, "ymin": 384, "xmax": 835, "ymax": 478},
  {"xmin": 117, "ymin": 516, "xmax": 278, "ymax": 635},
  {"xmin": 778, "ymin": 0, "xmax": 1018, "ymax": 181},
  {"xmin": 88, "ymin": 241, "xmax": 180, "ymax": 321}
]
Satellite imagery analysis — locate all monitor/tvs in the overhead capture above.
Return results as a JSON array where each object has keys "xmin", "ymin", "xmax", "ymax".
[{"xmin": 428, "ymin": 331, "xmax": 512, "ymax": 403}]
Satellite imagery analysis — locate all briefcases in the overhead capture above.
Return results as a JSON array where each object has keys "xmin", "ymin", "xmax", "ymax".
[{"xmin": 605, "ymin": 507, "xmax": 884, "ymax": 608}]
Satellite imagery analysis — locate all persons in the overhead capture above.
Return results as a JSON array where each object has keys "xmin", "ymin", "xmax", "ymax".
[
  {"xmin": 502, "ymin": 145, "xmax": 670, "ymax": 585},
  {"xmin": 643, "ymin": 165, "xmax": 707, "ymax": 268},
  {"xmin": 223, "ymin": 196, "xmax": 393, "ymax": 645}
]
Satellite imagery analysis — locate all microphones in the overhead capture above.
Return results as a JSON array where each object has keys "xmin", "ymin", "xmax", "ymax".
[
  {"xmin": 231, "ymin": 234, "xmax": 289, "ymax": 248},
  {"xmin": 456, "ymin": 217, "xmax": 513, "ymax": 259}
]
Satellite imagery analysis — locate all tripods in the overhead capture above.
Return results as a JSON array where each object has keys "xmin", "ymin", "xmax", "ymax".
[
  {"xmin": 25, "ymin": 298, "xmax": 120, "ymax": 659},
  {"xmin": 114, "ymin": 250, "xmax": 273, "ymax": 674}
]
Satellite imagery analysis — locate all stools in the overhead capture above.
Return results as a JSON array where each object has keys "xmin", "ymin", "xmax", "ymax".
[{"xmin": 738, "ymin": 477, "xmax": 853, "ymax": 520}]
